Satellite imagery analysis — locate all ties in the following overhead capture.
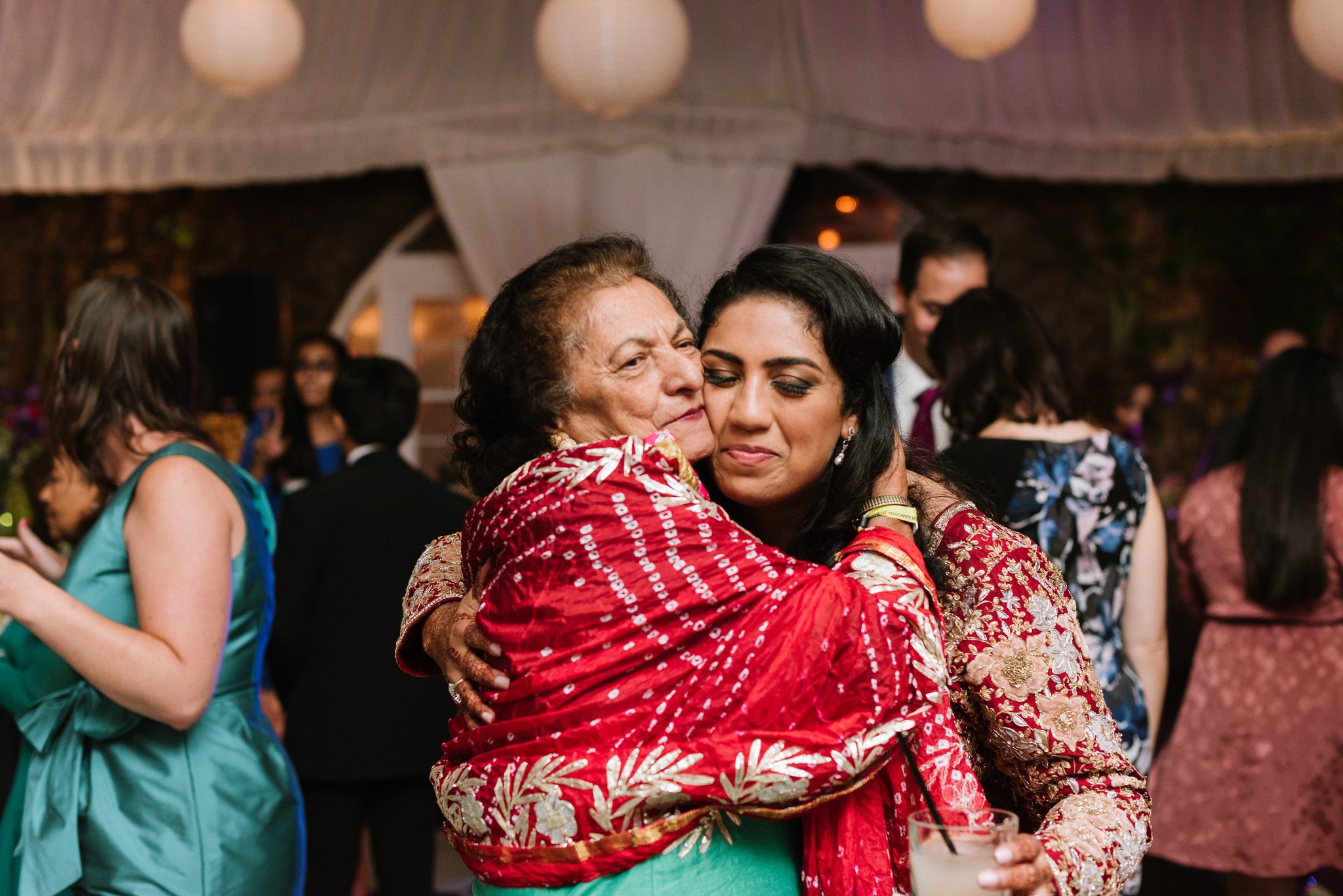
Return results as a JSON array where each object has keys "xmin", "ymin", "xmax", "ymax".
[{"xmin": 907, "ymin": 386, "xmax": 939, "ymax": 464}]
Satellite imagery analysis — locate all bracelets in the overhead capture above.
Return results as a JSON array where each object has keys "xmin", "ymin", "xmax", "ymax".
[
  {"xmin": 854, "ymin": 505, "xmax": 918, "ymax": 537},
  {"xmin": 852, "ymin": 494, "xmax": 914, "ymax": 528}
]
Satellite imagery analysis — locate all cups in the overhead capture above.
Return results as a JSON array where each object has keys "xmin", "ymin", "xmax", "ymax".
[{"xmin": 907, "ymin": 807, "xmax": 1018, "ymax": 896}]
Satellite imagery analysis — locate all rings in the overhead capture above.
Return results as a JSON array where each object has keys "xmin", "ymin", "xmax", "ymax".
[{"xmin": 446, "ymin": 677, "xmax": 468, "ymax": 704}]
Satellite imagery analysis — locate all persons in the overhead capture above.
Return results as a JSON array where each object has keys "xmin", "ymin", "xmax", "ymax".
[
  {"xmin": 231, "ymin": 364, "xmax": 290, "ymax": 481},
  {"xmin": 272, "ymin": 358, "xmax": 488, "ymax": 896},
  {"xmin": 1149, "ymin": 343, "xmax": 1342, "ymax": 896},
  {"xmin": 427, "ymin": 232, "xmax": 953, "ymax": 896},
  {"xmin": 910, "ymin": 287, "xmax": 1171, "ymax": 785},
  {"xmin": 2, "ymin": 272, "xmax": 312, "ymax": 896},
  {"xmin": 1160, "ymin": 321, "xmax": 1313, "ymax": 645},
  {"xmin": 865, "ymin": 216, "xmax": 1001, "ymax": 485},
  {"xmin": 393, "ymin": 244, "xmax": 1153, "ymax": 896},
  {"xmin": 28, "ymin": 436, "xmax": 119, "ymax": 565},
  {"xmin": 248, "ymin": 335, "xmax": 366, "ymax": 506}
]
